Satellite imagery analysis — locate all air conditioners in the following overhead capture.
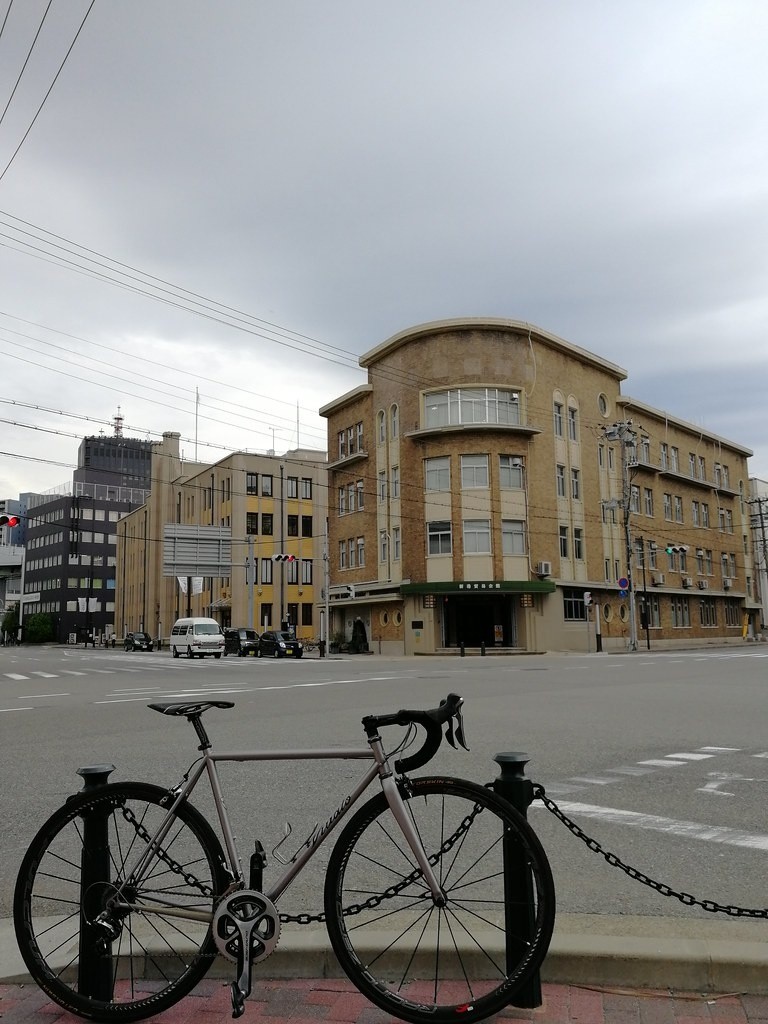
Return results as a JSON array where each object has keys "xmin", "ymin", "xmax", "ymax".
[
  {"xmin": 683, "ymin": 577, "xmax": 693, "ymax": 585},
  {"xmin": 653, "ymin": 572, "xmax": 666, "ymax": 583},
  {"xmin": 699, "ymin": 580, "xmax": 708, "ymax": 589},
  {"xmin": 724, "ymin": 579, "xmax": 732, "ymax": 587},
  {"xmin": 537, "ymin": 562, "xmax": 552, "ymax": 575}
]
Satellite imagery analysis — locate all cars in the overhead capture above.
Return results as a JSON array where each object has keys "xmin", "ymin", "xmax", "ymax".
[{"xmin": 260, "ymin": 630, "xmax": 303, "ymax": 660}]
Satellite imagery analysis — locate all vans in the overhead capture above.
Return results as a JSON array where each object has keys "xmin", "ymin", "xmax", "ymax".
[
  {"xmin": 224, "ymin": 628, "xmax": 264, "ymax": 657},
  {"xmin": 170, "ymin": 617, "xmax": 226, "ymax": 659},
  {"xmin": 124, "ymin": 632, "xmax": 154, "ymax": 652}
]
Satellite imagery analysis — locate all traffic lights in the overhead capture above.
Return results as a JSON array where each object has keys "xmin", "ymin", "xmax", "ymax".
[
  {"xmin": 0, "ymin": 513, "xmax": 21, "ymax": 528},
  {"xmin": 665, "ymin": 547, "xmax": 686, "ymax": 554},
  {"xmin": 274, "ymin": 554, "xmax": 296, "ymax": 563}
]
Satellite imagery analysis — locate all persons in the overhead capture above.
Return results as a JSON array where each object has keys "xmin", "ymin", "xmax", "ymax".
[
  {"xmin": 111, "ymin": 632, "xmax": 116, "ymax": 648},
  {"xmin": 7, "ymin": 632, "xmax": 16, "ymax": 647}
]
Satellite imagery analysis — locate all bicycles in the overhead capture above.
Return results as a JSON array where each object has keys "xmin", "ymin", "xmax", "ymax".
[{"xmin": 12, "ymin": 692, "xmax": 556, "ymax": 1024}]
